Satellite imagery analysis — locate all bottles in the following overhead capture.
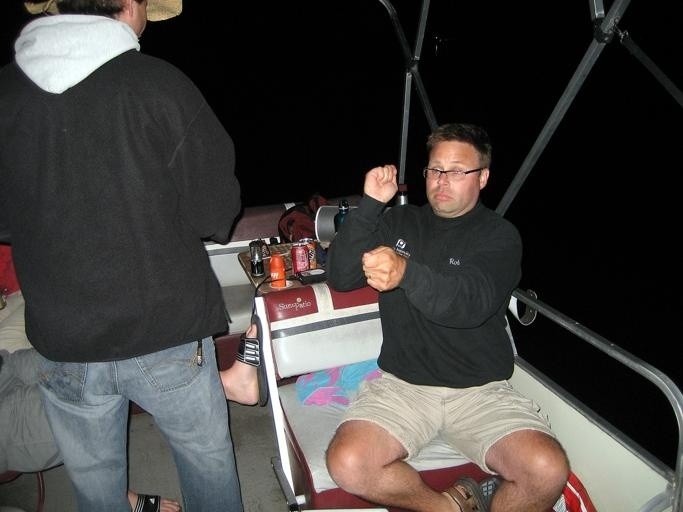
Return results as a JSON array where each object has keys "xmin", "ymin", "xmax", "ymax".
[
  {"xmin": 335, "ymin": 199, "xmax": 351, "ymax": 238},
  {"xmin": 397, "ymin": 184, "xmax": 407, "ymax": 207},
  {"xmin": 270, "ymin": 251, "xmax": 286, "ymax": 288}
]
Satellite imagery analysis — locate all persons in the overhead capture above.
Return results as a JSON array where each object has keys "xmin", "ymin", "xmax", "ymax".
[
  {"xmin": 0, "ymin": 313, "xmax": 270, "ymax": 511},
  {"xmin": 0, "ymin": 0, "xmax": 245, "ymax": 510},
  {"xmin": 321, "ymin": 121, "xmax": 571, "ymax": 511}
]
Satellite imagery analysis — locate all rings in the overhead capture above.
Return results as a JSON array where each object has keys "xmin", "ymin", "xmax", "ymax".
[{"xmin": 368, "ymin": 270, "xmax": 377, "ymax": 280}]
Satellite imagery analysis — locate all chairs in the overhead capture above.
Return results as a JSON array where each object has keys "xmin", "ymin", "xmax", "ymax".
[{"xmin": 254, "ymin": 282, "xmax": 598, "ymax": 512}]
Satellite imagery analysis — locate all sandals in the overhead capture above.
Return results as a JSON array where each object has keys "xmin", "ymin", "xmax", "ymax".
[{"xmin": 441, "ymin": 477, "xmax": 490, "ymax": 512}]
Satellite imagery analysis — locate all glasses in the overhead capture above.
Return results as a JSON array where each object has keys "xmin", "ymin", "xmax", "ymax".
[{"xmin": 422, "ymin": 167, "xmax": 486, "ymax": 182}]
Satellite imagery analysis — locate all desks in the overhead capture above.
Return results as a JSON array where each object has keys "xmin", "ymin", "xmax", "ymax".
[{"xmin": 237, "ymin": 242, "xmax": 329, "ymax": 295}]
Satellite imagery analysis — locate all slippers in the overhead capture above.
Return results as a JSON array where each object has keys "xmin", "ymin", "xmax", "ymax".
[
  {"xmin": 235, "ymin": 314, "xmax": 269, "ymax": 406},
  {"xmin": 132, "ymin": 494, "xmax": 161, "ymax": 512}
]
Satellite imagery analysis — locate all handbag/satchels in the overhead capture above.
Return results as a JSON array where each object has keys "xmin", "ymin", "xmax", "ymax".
[{"xmin": 279, "ymin": 194, "xmax": 330, "ymax": 243}]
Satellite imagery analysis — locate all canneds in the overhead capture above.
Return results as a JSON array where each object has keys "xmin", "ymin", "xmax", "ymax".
[
  {"xmin": 291, "ymin": 244, "xmax": 309, "ymax": 274},
  {"xmin": 304, "ymin": 241, "xmax": 317, "ymax": 269}
]
